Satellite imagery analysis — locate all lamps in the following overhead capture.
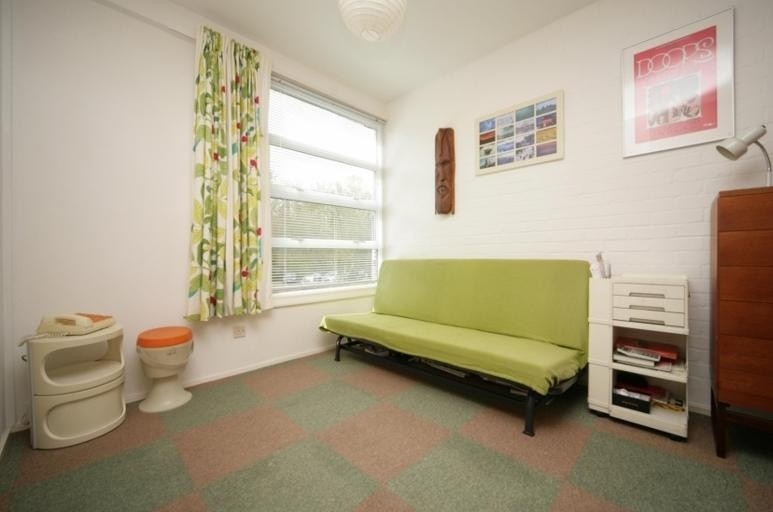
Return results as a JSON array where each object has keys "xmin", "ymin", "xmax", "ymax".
[{"xmin": 716, "ymin": 124, "xmax": 772, "ymax": 187}]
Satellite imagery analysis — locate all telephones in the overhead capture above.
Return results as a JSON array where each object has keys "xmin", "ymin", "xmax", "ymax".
[{"xmin": 38, "ymin": 313, "xmax": 114, "ymax": 335}]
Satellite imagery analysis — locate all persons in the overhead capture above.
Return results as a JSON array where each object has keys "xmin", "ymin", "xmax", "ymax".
[{"xmin": 432, "ymin": 130, "xmax": 453, "ymax": 214}]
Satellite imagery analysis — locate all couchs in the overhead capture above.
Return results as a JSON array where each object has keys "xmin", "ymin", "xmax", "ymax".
[{"xmin": 320, "ymin": 256, "xmax": 590, "ymax": 439}]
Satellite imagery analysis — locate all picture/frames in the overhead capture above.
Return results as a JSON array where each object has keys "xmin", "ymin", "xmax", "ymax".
[
  {"xmin": 624, "ymin": 7, "xmax": 734, "ymax": 158},
  {"xmin": 471, "ymin": 89, "xmax": 565, "ymax": 175}
]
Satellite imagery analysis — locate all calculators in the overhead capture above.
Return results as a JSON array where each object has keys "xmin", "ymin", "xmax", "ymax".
[{"xmin": 617, "ymin": 345, "xmax": 661, "ymax": 362}]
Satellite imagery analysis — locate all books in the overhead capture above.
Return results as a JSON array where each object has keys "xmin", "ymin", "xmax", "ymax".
[{"xmin": 611, "ymin": 342, "xmax": 682, "ymax": 414}]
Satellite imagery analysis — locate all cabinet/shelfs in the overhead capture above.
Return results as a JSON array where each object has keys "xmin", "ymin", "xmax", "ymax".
[
  {"xmin": 708, "ymin": 187, "xmax": 772, "ymax": 456},
  {"xmin": 25, "ymin": 324, "xmax": 126, "ymax": 452},
  {"xmin": 588, "ymin": 273, "xmax": 693, "ymax": 442}
]
